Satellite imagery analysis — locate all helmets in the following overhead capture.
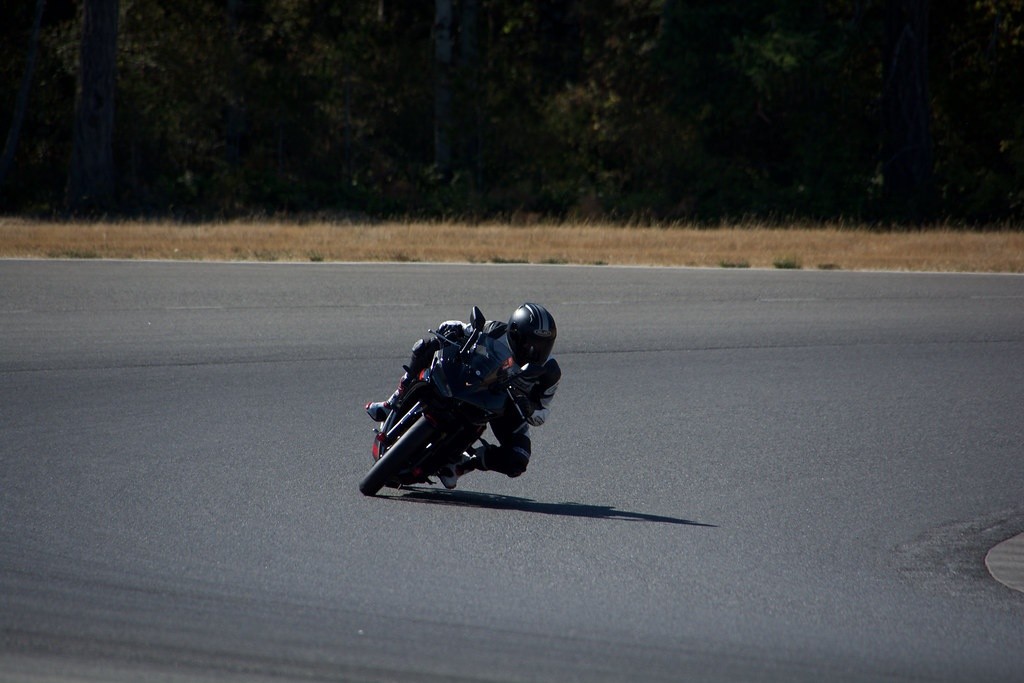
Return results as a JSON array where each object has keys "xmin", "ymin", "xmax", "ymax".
[{"xmin": 506, "ymin": 302, "xmax": 557, "ymax": 373}]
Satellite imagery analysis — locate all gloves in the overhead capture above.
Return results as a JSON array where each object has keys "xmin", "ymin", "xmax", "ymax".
[
  {"xmin": 440, "ymin": 325, "xmax": 462, "ymax": 346},
  {"xmin": 515, "ymin": 394, "xmax": 533, "ymax": 418}
]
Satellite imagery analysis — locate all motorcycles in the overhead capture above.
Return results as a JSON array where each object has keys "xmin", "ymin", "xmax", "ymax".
[{"xmin": 359, "ymin": 305, "xmax": 562, "ymax": 498}]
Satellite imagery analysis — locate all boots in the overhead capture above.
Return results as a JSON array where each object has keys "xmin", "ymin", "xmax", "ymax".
[
  {"xmin": 438, "ymin": 444, "xmax": 497, "ymax": 489},
  {"xmin": 366, "ymin": 375, "xmax": 410, "ymax": 421}
]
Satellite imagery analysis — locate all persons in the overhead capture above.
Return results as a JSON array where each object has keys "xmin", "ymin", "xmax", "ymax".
[{"xmin": 364, "ymin": 303, "xmax": 562, "ymax": 489}]
